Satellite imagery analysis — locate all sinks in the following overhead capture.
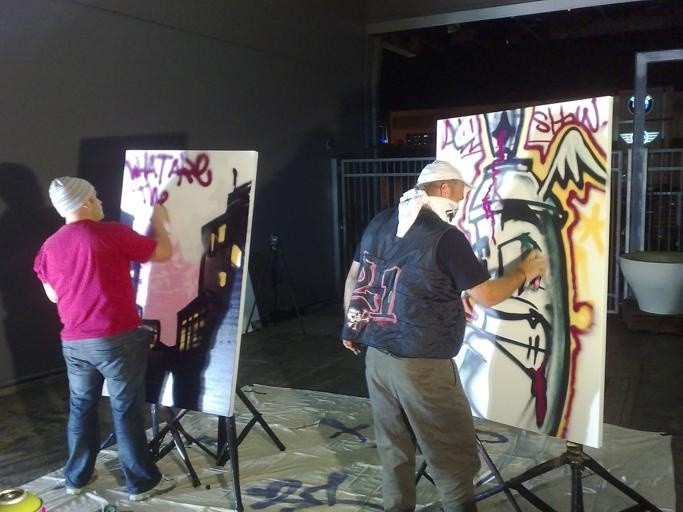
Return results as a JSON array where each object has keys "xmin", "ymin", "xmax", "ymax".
[{"xmin": 619, "ymin": 251, "xmax": 683, "ymax": 317}]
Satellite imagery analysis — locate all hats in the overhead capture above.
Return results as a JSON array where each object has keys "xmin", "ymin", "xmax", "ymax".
[
  {"xmin": 415, "ymin": 160, "xmax": 475, "ymax": 189},
  {"xmin": 48, "ymin": 176, "xmax": 95, "ymax": 218}
]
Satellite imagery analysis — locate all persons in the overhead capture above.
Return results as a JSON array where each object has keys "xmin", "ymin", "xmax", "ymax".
[
  {"xmin": 342, "ymin": 161, "xmax": 548, "ymax": 512},
  {"xmin": 32, "ymin": 176, "xmax": 177, "ymax": 501}
]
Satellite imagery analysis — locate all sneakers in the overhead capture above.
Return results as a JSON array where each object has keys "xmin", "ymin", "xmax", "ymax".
[
  {"xmin": 129, "ymin": 474, "xmax": 177, "ymax": 501},
  {"xmin": 66, "ymin": 468, "xmax": 97, "ymax": 495}
]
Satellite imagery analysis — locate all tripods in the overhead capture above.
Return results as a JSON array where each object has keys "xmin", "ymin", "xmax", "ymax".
[{"xmin": 245, "ymin": 251, "xmax": 307, "ymax": 337}]
